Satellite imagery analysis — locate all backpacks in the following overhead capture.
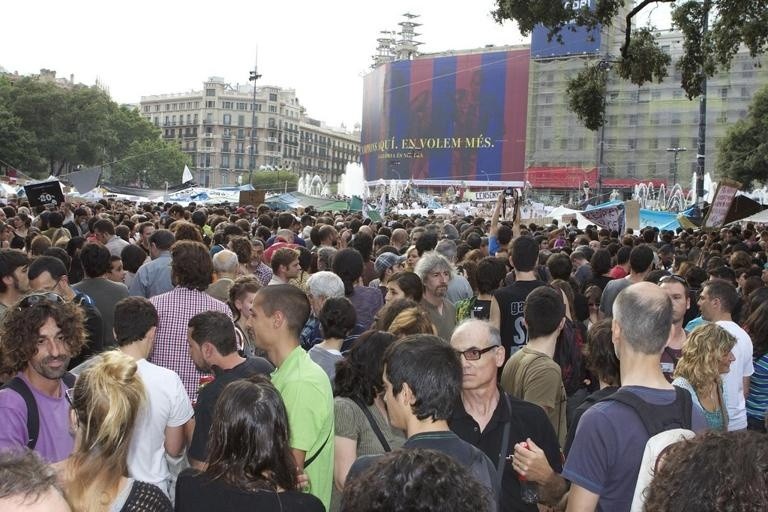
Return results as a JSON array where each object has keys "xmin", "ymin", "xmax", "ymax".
[{"xmin": 597, "ymin": 385, "xmax": 698, "ymax": 512}]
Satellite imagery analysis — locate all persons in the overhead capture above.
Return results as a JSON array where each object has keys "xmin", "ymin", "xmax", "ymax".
[{"xmin": 1, "ymin": 183, "xmax": 768, "ymax": 512}]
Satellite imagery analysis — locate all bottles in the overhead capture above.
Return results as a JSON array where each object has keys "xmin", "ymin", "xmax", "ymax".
[{"xmin": 516, "ymin": 441, "xmax": 539, "ymax": 504}]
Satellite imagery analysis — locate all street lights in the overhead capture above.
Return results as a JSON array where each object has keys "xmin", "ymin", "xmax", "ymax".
[
  {"xmin": 665, "ymin": 147, "xmax": 687, "ymax": 191},
  {"xmin": 392, "ymin": 168, "xmax": 402, "ymax": 183},
  {"xmin": 481, "ymin": 170, "xmax": 490, "ymax": 192},
  {"xmin": 248, "ymin": 68, "xmax": 263, "ymax": 183},
  {"xmin": 589, "ymin": 58, "xmax": 622, "ymax": 200}
]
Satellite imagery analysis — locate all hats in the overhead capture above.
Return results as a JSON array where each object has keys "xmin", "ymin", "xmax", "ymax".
[{"xmin": 373, "ymin": 252, "xmax": 408, "ymax": 277}]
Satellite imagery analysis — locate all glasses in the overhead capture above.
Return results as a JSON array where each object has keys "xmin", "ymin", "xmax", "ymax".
[
  {"xmin": 658, "ymin": 275, "xmax": 689, "ymax": 287},
  {"xmin": 458, "ymin": 344, "xmax": 499, "ymax": 360},
  {"xmin": 16, "ymin": 293, "xmax": 66, "ymax": 309}
]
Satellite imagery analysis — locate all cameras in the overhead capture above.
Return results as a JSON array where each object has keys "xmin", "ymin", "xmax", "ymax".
[{"xmin": 504, "ymin": 188, "xmax": 514, "ymax": 195}]
